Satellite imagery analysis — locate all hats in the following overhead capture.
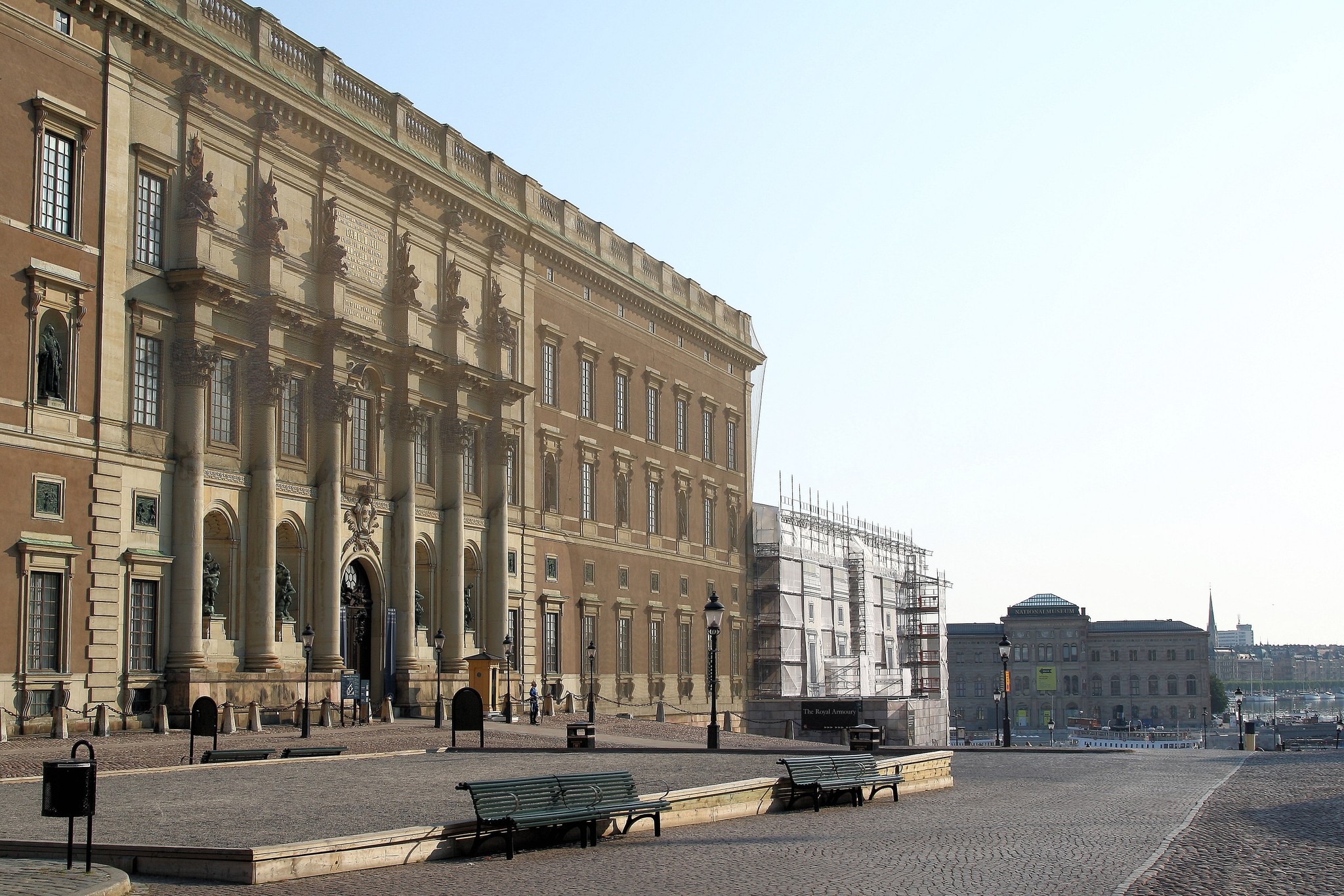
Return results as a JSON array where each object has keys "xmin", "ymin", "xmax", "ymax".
[{"xmin": 531, "ymin": 679, "xmax": 537, "ymax": 686}]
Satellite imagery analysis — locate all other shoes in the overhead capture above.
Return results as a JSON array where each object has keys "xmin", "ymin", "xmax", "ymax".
[
  {"xmin": 530, "ymin": 722, "xmax": 536, "ymax": 724},
  {"xmin": 534, "ymin": 721, "xmax": 539, "ymax": 723}
]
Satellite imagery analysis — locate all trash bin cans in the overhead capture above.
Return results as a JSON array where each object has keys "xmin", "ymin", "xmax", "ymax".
[
  {"xmin": 850, "ymin": 724, "xmax": 879, "ymax": 750},
  {"xmin": 567, "ymin": 722, "xmax": 595, "ymax": 748},
  {"xmin": 40, "ymin": 757, "xmax": 97, "ymax": 818}
]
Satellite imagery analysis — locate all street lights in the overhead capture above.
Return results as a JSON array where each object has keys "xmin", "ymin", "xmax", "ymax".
[
  {"xmin": 587, "ymin": 642, "xmax": 598, "ymax": 724},
  {"xmin": 951, "ymin": 714, "xmax": 961, "ymax": 745},
  {"xmin": 1047, "ymin": 718, "xmax": 1055, "ymax": 747},
  {"xmin": 502, "ymin": 633, "xmax": 514, "ymax": 724},
  {"xmin": 998, "ymin": 633, "xmax": 1014, "ymax": 747},
  {"xmin": 1235, "ymin": 687, "xmax": 1244, "ymax": 750},
  {"xmin": 1202, "ymin": 711, "xmax": 1209, "ymax": 749},
  {"xmin": 300, "ymin": 622, "xmax": 316, "ymax": 737},
  {"xmin": 993, "ymin": 689, "xmax": 1001, "ymax": 745},
  {"xmin": 1335, "ymin": 720, "xmax": 1344, "ymax": 750},
  {"xmin": 704, "ymin": 592, "xmax": 726, "ymax": 748},
  {"xmin": 434, "ymin": 626, "xmax": 445, "ymax": 727}
]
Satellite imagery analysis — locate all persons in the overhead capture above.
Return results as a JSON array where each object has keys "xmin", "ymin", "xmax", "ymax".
[
  {"xmin": 39, "ymin": 324, "xmax": 66, "ymax": 401},
  {"xmin": 203, "ymin": 552, "xmax": 221, "ymax": 606},
  {"xmin": 464, "ymin": 583, "xmax": 475, "ymax": 627},
  {"xmin": 529, "ymin": 679, "xmax": 539, "ymax": 724},
  {"xmin": 276, "ymin": 562, "xmax": 296, "ymax": 617},
  {"xmin": 415, "ymin": 591, "xmax": 424, "ymax": 625}
]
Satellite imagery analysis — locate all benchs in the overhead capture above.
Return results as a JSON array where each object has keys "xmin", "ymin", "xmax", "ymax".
[
  {"xmin": 455, "ymin": 769, "xmax": 672, "ymax": 860},
  {"xmin": 269, "ymin": 746, "xmax": 349, "ymax": 758},
  {"xmin": 776, "ymin": 752, "xmax": 905, "ymax": 812},
  {"xmin": 181, "ymin": 749, "xmax": 277, "ymax": 764}
]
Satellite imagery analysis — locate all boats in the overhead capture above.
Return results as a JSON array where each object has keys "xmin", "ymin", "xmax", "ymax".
[
  {"xmin": 1053, "ymin": 711, "xmax": 1205, "ymax": 749},
  {"xmin": 1224, "ymin": 670, "xmax": 1344, "ymax": 701},
  {"xmin": 1249, "ymin": 708, "xmax": 1344, "ymax": 740}
]
